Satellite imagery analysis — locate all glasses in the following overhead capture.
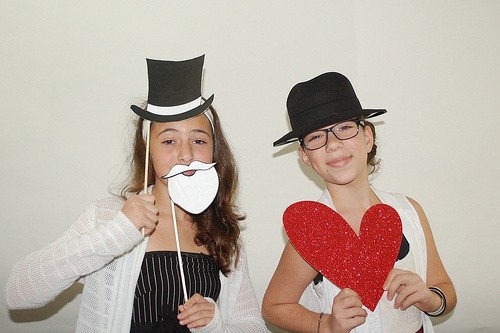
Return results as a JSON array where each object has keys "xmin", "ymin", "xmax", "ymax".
[{"xmin": 297, "ymin": 119, "xmax": 365, "ymax": 151}]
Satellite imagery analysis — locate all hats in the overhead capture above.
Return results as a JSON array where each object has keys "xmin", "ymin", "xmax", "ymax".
[
  {"xmin": 129, "ymin": 54, "xmax": 214, "ymax": 122},
  {"xmin": 273, "ymin": 72, "xmax": 387, "ymax": 147}
]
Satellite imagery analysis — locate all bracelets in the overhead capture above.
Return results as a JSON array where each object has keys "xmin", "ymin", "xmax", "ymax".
[
  {"xmin": 424, "ymin": 287, "xmax": 446, "ymax": 317},
  {"xmin": 317, "ymin": 312, "xmax": 324, "ymax": 333}
]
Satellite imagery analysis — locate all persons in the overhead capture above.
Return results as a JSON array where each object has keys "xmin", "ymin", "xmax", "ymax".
[
  {"xmin": 262, "ymin": 71, "xmax": 457, "ymax": 333},
  {"xmin": 5, "ymin": 54, "xmax": 271, "ymax": 333}
]
